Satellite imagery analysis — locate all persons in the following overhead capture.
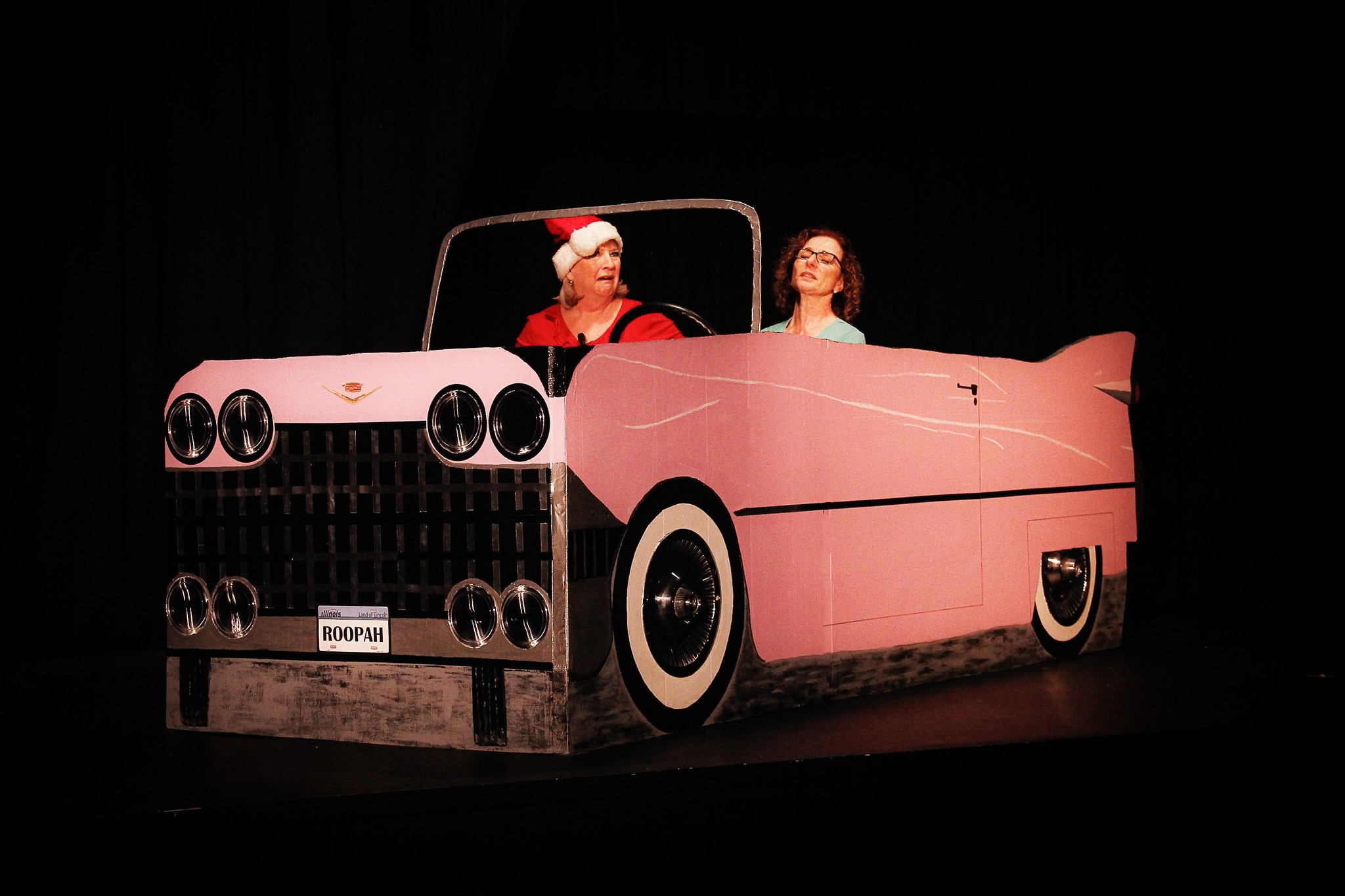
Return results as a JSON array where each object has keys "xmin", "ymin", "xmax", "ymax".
[
  {"xmin": 760, "ymin": 228, "xmax": 866, "ymax": 345},
  {"xmin": 515, "ymin": 215, "xmax": 686, "ymax": 348}
]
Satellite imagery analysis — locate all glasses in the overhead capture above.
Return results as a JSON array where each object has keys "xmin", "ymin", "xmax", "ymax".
[{"xmin": 794, "ymin": 248, "xmax": 844, "ymax": 273}]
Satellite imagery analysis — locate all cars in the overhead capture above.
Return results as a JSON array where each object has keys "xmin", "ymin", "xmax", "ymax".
[{"xmin": 162, "ymin": 196, "xmax": 1136, "ymax": 756}]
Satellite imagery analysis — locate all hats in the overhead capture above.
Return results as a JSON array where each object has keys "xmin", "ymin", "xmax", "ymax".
[{"xmin": 545, "ymin": 214, "xmax": 623, "ymax": 282}]
